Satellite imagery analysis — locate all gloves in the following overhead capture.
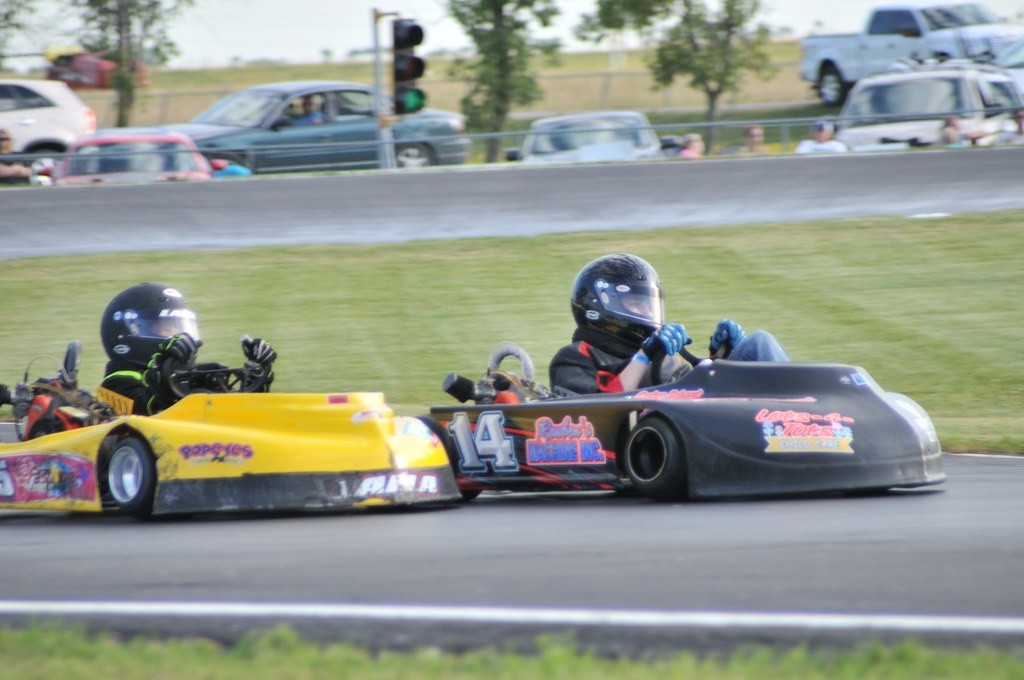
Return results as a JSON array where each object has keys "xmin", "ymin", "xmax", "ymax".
[
  {"xmin": 640, "ymin": 322, "xmax": 693, "ymax": 362},
  {"xmin": 157, "ymin": 332, "xmax": 202, "ymax": 365},
  {"xmin": 241, "ymin": 336, "xmax": 277, "ymax": 384},
  {"xmin": 709, "ymin": 318, "xmax": 746, "ymax": 360}
]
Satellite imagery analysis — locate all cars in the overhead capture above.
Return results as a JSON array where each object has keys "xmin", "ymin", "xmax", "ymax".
[
  {"xmin": 32, "ymin": 133, "xmax": 228, "ymax": 186},
  {"xmin": 0, "ymin": 79, "xmax": 96, "ymax": 152},
  {"xmin": 47, "ymin": 51, "xmax": 148, "ymax": 89},
  {"xmin": 833, "ymin": 70, "xmax": 1024, "ymax": 149},
  {"xmin": 97, "ymin": 81, "xmax": 470, "ymax": 175},
  {"xmin": 505, "ymin": 112, "xmax": 683, "ymax": 163}
]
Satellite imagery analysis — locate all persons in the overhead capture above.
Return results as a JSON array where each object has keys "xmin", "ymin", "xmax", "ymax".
[
  {"xmin": 100, "ymin": 282, "xmax": 278, "ymax": 417},
  {"xmin": 549, "ymin": 253, "xmax": 746, "ymax": 398},
  {"xmin": 0, "ymin": 127, "xmax": 30, "ymax": 179},
  {"xmin": 738, "ymin": 125, "xmax": 770, "ymax": 155},
  {"xmin": 675, "ymin": 133, "xmax": 706, "ymax": 159},
  {"xmin": 942, "ymin": 107, "xmax": 1024, "ymax": 148},
  {"xmin": 271, "ymin": 93, "xmax": 330, "ymax": 125},
  {"xmin": 795, "ymin": 121, "xmax": 846, "ymax": 155}
]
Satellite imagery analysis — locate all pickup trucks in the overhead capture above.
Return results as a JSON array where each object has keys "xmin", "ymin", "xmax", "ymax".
[{"xmin": 799, "ymin": 2, "xmax": 1024, "ymax": 109}]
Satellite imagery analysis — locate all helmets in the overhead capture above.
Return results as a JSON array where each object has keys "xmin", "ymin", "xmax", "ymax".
[
  {"xmin": 570, "ymin": 253, "xmax": 666, "ymax": 348},
  {"xmin": 100, "ymin": 283, "xmax": 203, "ymax": 366}
]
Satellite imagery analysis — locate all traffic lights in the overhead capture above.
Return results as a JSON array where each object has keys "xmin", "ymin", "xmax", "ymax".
[{"xmin": 393, "ymin": 19, "xmax": 426, "ymax": 115}]
